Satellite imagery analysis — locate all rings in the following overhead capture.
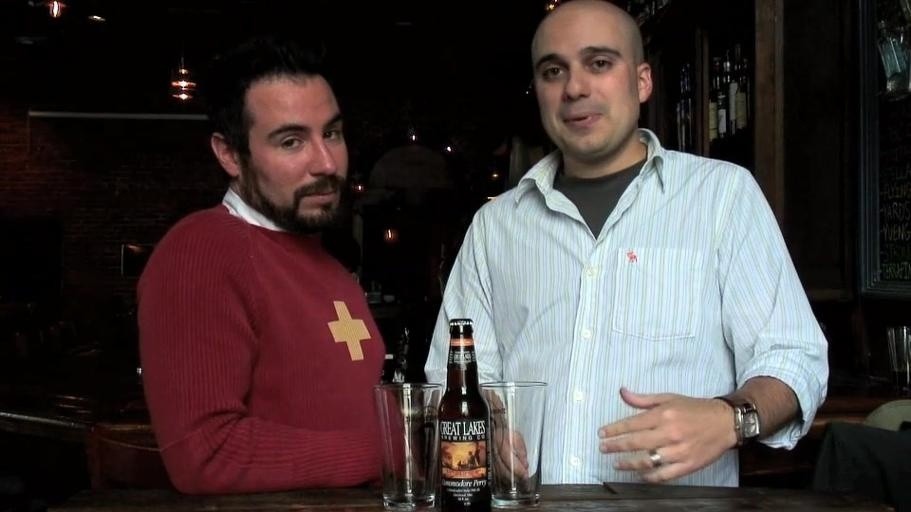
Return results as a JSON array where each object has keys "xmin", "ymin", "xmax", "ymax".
[{"xmin": 648, "ymin": 449, "xmax": 662, "ymax": 468}]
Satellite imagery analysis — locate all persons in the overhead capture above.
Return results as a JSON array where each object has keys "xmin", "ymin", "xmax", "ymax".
[
  {"xmin": 424, "ymin": 0, "xmax": 830, "ymax": 486},
  {"xmin": 136, "ymin": 48, "xmax": 423, "ymax": 496}
]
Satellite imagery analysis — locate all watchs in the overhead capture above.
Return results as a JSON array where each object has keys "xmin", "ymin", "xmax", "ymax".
[{"xmin": 712, "ymin": 391, "xmax": 759, "ymax": 448}]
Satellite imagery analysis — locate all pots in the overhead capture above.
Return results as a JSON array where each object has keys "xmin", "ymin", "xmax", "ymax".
[{"xmin": 0, "ymin": 405, "xmax": 170, "ymax": 490}]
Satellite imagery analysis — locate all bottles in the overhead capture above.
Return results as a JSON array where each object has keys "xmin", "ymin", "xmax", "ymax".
[
  {"xmin": 437, "ymin": 318, "xmax": 489, "ymax": 509},
  {"xmin": 675, "ymin": 42, "xmax": 753, "ymax": 153},
  {"xmin": 875, "ymin": 17, "xmax": 908, "ymax": 92}
]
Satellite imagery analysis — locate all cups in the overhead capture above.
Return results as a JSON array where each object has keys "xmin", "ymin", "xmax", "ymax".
[
  {"xmin": 479, "ymin": 380, "xmax": 550, "ymax": 509},
  {"xmin": 371, "ymin": 383, "xmax": 444, "ymax": 510},
  {"xmin": 887, "ymin": 325, "xmax": 910, "ymax": 397}
]
